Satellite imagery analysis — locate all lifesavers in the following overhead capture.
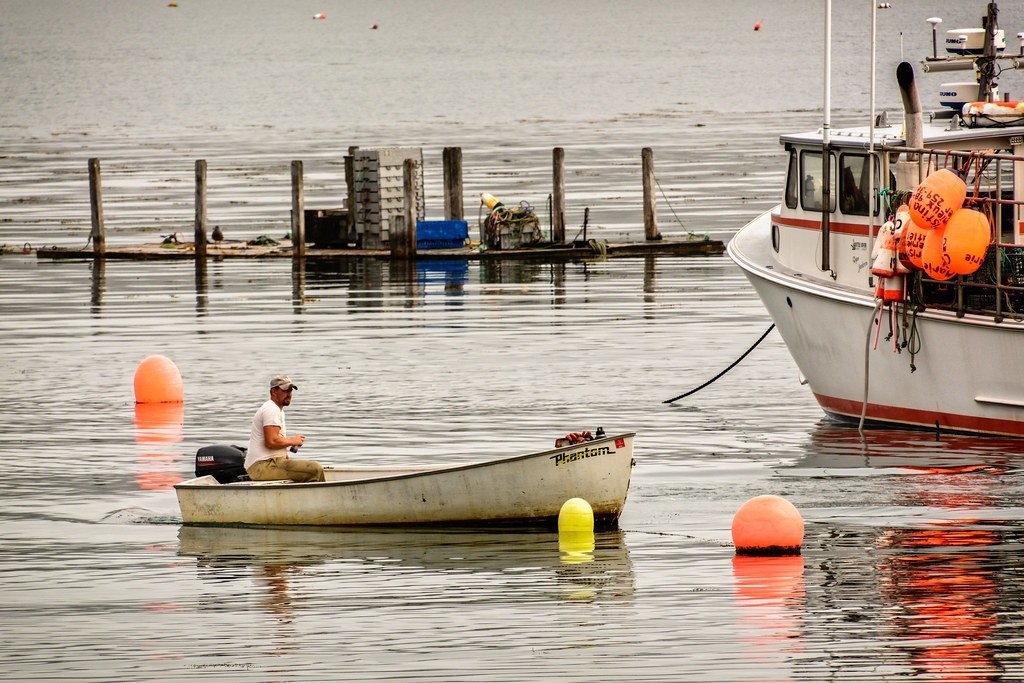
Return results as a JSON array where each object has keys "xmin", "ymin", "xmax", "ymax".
[{"xmin": 959, "ymin": 99, "xmax": 1024, "ymax": 127}]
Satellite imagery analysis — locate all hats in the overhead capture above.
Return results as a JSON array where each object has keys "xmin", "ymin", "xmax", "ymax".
[{"xmin": 270, "ymin": 375, "xmax": 298, "ymax": 390}]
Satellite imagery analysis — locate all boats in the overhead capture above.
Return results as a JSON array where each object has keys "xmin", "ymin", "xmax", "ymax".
[
  {"xmin": 171, "ymin": 425, "xmax": 636, "ymax": 530},
  {"xmin": 725, "ymin": 0, "xmax": 1023, "ymax": 440}
]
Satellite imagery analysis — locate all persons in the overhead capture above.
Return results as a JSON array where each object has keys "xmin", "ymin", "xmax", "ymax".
[{"xmin": 243, "ymin": 376, "xmax": 326, "ymax": 482}]
